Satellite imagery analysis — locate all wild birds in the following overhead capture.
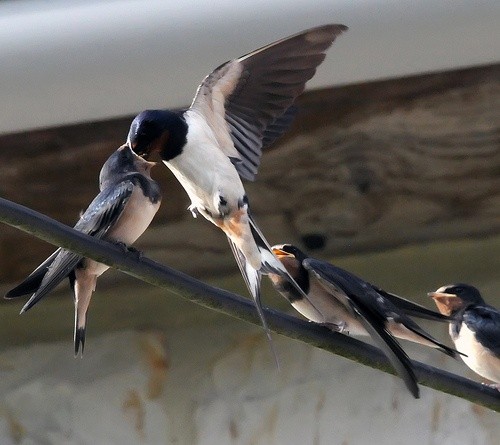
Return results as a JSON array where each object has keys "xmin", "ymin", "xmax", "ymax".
[
  {"xmin": 265, "ymin": 244, "xmax": 469, "ymax": 399},
  {"xmin": 5, "ymin": 141, "xmax": 162, "ymax": 359},
  {"xmin": 426, "ymin": 283, "xmax": 500, "ymax": 392},
  {"xmin": 126, "ymin": 22, "xmax": 349, "ymax": 371}
]
